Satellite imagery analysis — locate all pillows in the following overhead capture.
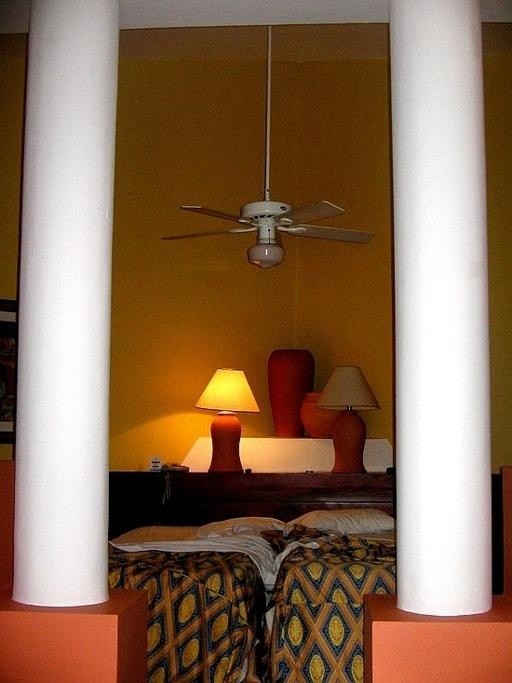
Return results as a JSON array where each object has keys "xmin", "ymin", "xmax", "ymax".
[
  {"xmin": 288, "ymin": 509, "xmax": 394, "ymax": 534},
  {"xmin": 200, "ymin": 516, "xmax": 285, "ymax": 537}
]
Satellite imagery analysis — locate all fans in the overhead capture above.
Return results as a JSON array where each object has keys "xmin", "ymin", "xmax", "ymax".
[{"xmin": 158, "ymin": 25, "xmax": 376, "ymax": 242}]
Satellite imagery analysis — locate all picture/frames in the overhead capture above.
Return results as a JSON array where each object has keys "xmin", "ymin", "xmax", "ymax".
[{"xmin": 0, "ymin": 298, "xmax": 18, "ymax": 444}]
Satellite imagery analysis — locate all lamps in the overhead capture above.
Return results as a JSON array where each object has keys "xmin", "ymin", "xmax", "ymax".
[
  {"xmin": 317, "ymin": 366, "xmax": 381, "ymax": 474},
  {"xmin": 248, "ymin": 218, "xmax": 285, "ymax": 267},
  {"xmin": 194, "ymin": 366, "xmax": 260, "ymax": 475}
]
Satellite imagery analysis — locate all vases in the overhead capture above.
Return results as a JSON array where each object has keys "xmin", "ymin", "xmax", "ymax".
[
  {"xmin": 270, "ymin": 349, "xmax": 314, "ymax": 440},
  {"xmin": 301, "ymin": 391, "xmax": 337, "ymax": 438}
]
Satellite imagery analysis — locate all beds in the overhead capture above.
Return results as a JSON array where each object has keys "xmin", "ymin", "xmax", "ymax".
[
  {"xmin": 109, "ymin": 524, "xmax": 269, "ymax": 683},
  {"xmin": 277, "ymin": 531, "xmax": 398, "ymax": 683}
]
